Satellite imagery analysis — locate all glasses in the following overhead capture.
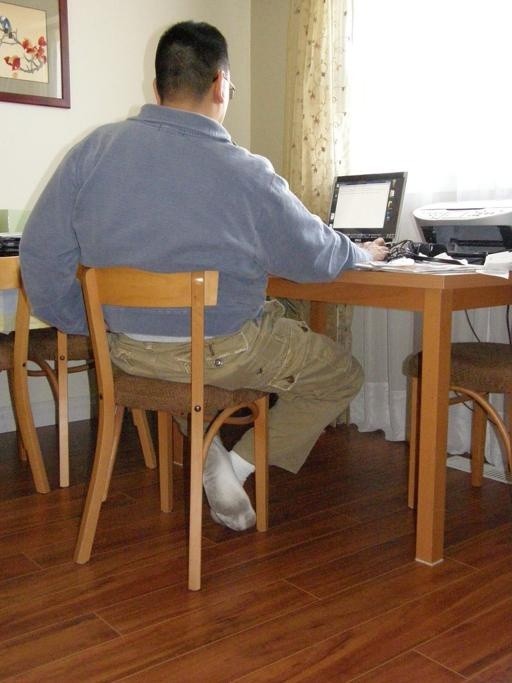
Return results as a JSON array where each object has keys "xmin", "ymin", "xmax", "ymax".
[{"xmin": 225, "ymin": 75, "xmax": 236, "ymax": 99}]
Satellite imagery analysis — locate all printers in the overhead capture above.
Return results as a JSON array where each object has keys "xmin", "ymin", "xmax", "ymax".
[{"xmin": 413, "ymin": 201, "xmax": 512, "ymax": 264}]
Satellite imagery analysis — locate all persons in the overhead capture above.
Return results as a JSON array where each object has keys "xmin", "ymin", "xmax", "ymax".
[{"xmin": 18, "ymin": 21, "xmax": 390, "ymax": 532}]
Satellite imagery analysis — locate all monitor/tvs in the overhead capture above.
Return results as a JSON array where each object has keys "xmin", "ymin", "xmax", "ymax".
[{"xmin": 327, "ymin": 170, "xmax": 407, "ymax": 249}]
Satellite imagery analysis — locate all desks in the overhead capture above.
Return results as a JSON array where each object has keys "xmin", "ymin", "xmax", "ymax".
[{"xmin": 172, "ymin": 259, "xmax": 512, "ymax": 569}]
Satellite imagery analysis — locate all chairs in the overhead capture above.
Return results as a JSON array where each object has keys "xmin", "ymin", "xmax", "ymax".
[
  {"xmin": 5, "ymin": 328, "xmax": 155, "ymax": 489},
  {"xmin": 72, "ymin": 260, "xmax": 272, "ymax": 592},
  {"xmin": 0, "ymin": 251, "xmax": 52, "ymax": 497},
  {"xmin": 402, "ymin": 340, "xmax": 511, "ymax": 513}
]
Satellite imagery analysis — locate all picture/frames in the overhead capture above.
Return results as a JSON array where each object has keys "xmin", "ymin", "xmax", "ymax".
[{"xmin": 0, "ymin": 0, "xmax": 72, "ymax": 110}]
